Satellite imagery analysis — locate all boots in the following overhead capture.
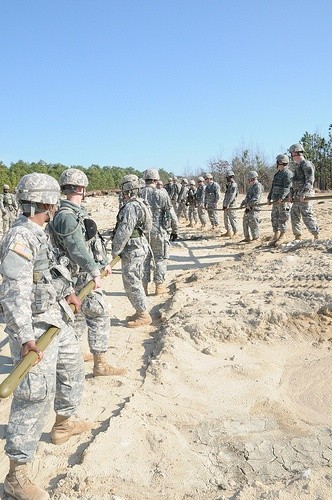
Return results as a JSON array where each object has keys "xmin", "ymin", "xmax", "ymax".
[
  {"xmin": 155, "ymin": 283, "xmax": 170, "ymax": 295},
  {"xmin": 93, "ymin": 353, "xmax": 127, "ymax": 376},
  {"xmin": 126, "ymin": 310, "xmax": 139, "ymax": 321},
  {"xmin": 83, "ymin": 353, "xmax": 93, "ymax": 362},
  {"xmin": 51, "ymin": 415, "xmax": 96, "ymax": 445},
  {"xmin": 142, "ymin": 284, "xmax": 149, "ymax": 296},
  {"xmin": 4, "ymin": 460, "xmax": 50, "ymax": 500},
  {"xmin": 127, "ymin": 310, "xmax": 152, "ymax": 328},
  {"xmin": 177, "ymin": 218, "xmax": 320, "ymax": 246}
]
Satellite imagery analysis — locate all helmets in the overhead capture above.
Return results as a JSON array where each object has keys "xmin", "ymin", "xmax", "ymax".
[
  {"xmin": 182, "ymin": 178, "xmax": 188, "ymax": 184},
  {"xmin": 121, "ymin": 174, "xmax": 140, "ymax": 192},
  {"xmin": 197, "ymin": 176, "xmax": 205, "ymax": 183},
  {"xmin": 138, "ymin": 178, "xmax": 145, "ymax": 184},
  {"xmin": 59, "ymin": 168, "xmax": 89, "ymax": 187},
  {"xmin": 276, "ymin": 154, "xmax": 289, "ymax": 163},
  {"xmin": 225, "ymin": 171, "xmax": 235, "ymax": 177},
  {"xmin": 168, "ymin": 178, "xmax": 173, "ymax": 182},
  {"xmin": 143, "ymin": 168, "xmax": 160, "ymax": 180},
  {"xmin": 173, "ymin": 176, "xmax": 177, "ymax": 180},
  {"xmin": 289, "ymin": 144, "xmax": 305, "ymax": 153},
  {"xmin": 205, "ymin": 173, "xmax": 213, "ymax": 179},
  {"xmin": 157, "ymin": 181, "xmax": 163, "ymax": 185},
  {"xmin": 247, "ymin": 171, "xmax": 259, "ymax": 180},
  {"xmin": 16, "ymin": 173, "xmax": 61, "ymax": 204},
  {"xmin": 189, "ymin": 180, "xmax": 196, "ymax": 185},
  {"xmin": 3, "ymin": 184, "xmax": 9, "ymax": 190}
]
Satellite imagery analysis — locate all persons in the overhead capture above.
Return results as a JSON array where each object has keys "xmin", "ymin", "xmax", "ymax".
[
  {"xmin": 156, "ymin": 171, "xmax": 220, "ymax": 232},
  {"xmin": 221, "ymin": 170, "xmax": 238, "ymax": 237},
  {"xmin": 284, "ymin": 145, "xmax": 322, "ymax": 242},
  {"xmin": 51, "ymin": 169, "xmax": 130, "ymax": 376},
  {"xmin": 112, "ymin": 174, "xmax": 155, "ymax": 327},
  {"xmin": 0, "ymin": 173, "xmax": 95, "ymax": 500},
  {"xmin": 139, "ymin": 169, "xmax": 182, "ymax": 293},
  {"xmin": 266, "ymin": 154, "xmax": 291, "ymax": 244},
  {"xmin": 240, "ymin": 170, "xmax": 262, "ymax": 244},
  {"xmin": 1, "ymin": 181, "xmax": 21, "ymax": 235}
]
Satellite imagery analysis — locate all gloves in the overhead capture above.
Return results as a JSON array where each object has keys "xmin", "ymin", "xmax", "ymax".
[{"xmin": 169, "ymin": 233, "xmax": 177, "ymax": 242}]
